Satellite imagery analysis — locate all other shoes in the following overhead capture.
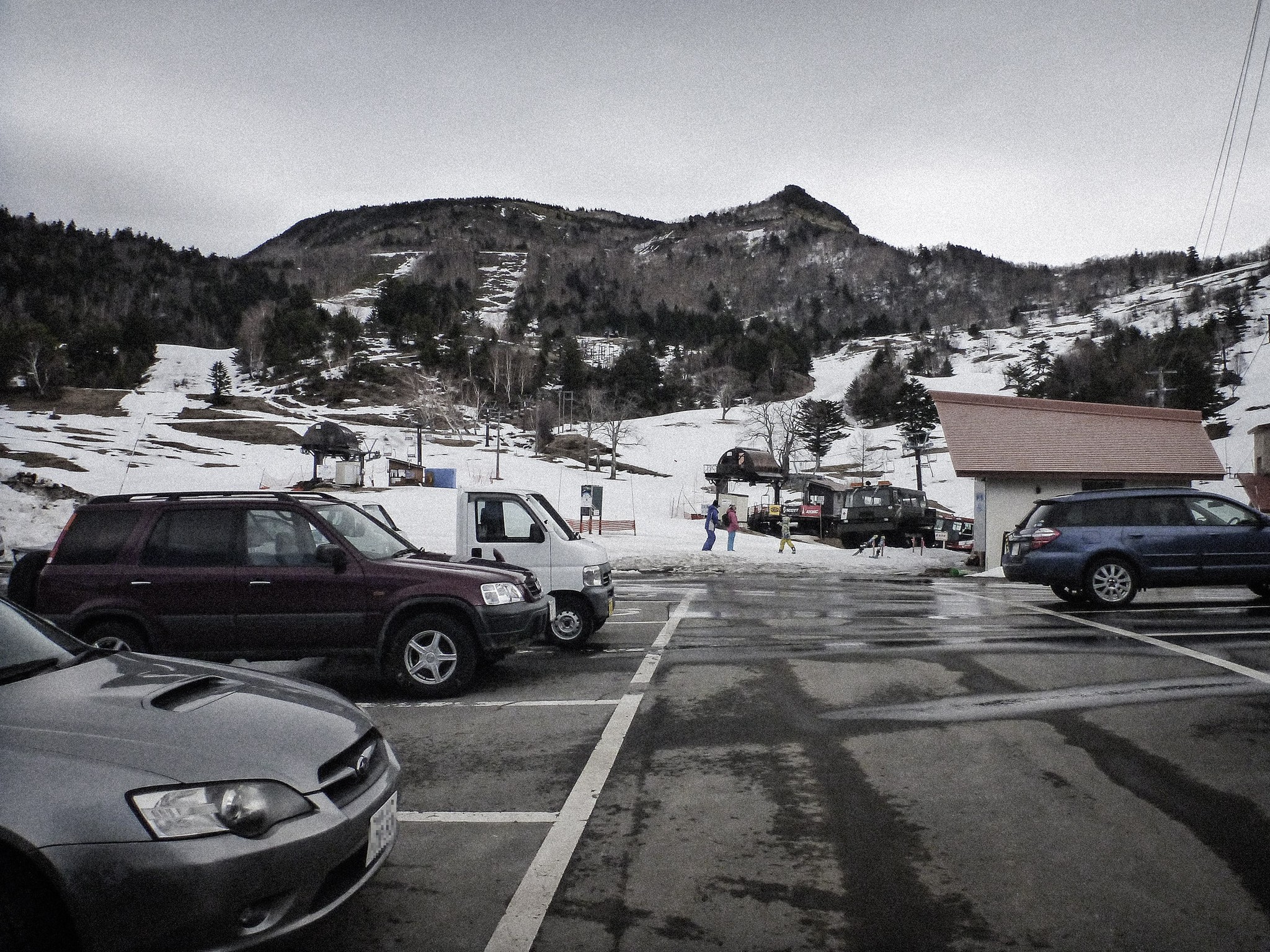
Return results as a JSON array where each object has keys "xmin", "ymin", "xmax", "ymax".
[{"xmin": 728, "ymin": 550, "xmax": 735, "ymax": 551}]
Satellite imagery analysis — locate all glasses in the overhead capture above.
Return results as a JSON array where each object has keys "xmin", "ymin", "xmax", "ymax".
[{"xmin": 729, "ymin": 503, "xmax": 735, "ymax": 509}]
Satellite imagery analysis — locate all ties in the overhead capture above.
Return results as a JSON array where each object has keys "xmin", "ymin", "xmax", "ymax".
[{"xmin": 729, "ymin": 504, "xmax": 736, "ymax": 510}]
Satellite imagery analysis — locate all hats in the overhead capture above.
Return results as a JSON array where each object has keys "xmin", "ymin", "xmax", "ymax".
[
  {"xmin": 713, "ymin": 500, "xmax": 718, "ymax": 506},
  {"xmin": 783, "ymin": 517, "xmax": 790, "ymax": 522}
]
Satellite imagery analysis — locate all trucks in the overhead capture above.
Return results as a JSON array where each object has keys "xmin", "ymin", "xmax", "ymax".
[{"xmin": 287, "ymin": 485, "xmax": 618, "ymax": 653}]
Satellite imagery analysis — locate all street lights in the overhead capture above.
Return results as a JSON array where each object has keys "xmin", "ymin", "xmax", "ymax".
[{"xmin": 903, "ymin": 428, "xmax": 931, "ymax": 492}]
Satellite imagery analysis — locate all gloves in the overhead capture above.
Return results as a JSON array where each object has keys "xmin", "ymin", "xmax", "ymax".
[{"xmin": 784, "ymin": 533, "xmax": 790, "ymax": 539}]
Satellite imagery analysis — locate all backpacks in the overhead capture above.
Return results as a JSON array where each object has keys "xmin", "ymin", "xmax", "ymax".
[{"xmin": 722, "ymin": 511, "xmax": 734, "ymax": 527}]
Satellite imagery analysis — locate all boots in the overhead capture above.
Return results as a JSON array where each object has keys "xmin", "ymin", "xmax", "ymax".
[
  {"xmin": 791, "ymin": 547, "xmax": 795, "ymax": 554},
  {"xmin": 778, "ymin": 549, "xmax": 783, "ymax": 553}
]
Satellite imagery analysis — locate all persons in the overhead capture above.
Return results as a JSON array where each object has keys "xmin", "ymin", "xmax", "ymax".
[
  {"xmin": 725, "ymin": 504, "xmax": 738, "ymax": 551},
  {"xmin": 777, "ymin": 516, "xmax": 796, "ymax": 554},
  {"xmin": 701, "ymin": 499, "xmax": 722, "ymax": 551}
]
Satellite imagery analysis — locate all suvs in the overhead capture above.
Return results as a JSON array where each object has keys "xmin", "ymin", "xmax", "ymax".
[
  {"xmin": 1001, "ymin": 486, "xmax": 1270, "ymax": 610},
  {"xmin": 6, "ymin": 486, "xmax": 550, "ymax": 703}
]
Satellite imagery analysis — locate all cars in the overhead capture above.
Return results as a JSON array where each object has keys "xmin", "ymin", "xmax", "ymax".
[{"xmin": 1, "ymin": 598, "xmax": 403, "ymax": 952}]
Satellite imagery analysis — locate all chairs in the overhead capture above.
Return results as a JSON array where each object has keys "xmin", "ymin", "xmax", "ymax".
[
  {"xmin": 247, "ymin": 527, "xmax": 273, "ymax": 566},
  {"xmin": 481, "ymin": 508, "xmax": 502, "ymax": 538},
  {"xmin": 275, "ymin": 532, "xmax": 300, "ymax": 567}
]
